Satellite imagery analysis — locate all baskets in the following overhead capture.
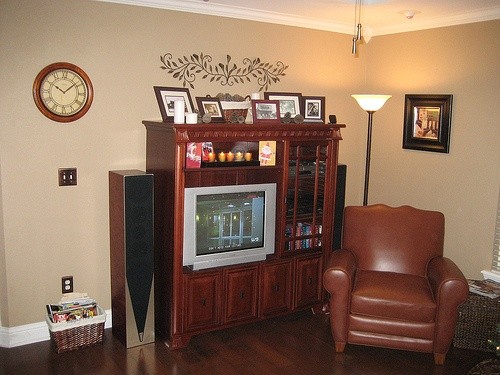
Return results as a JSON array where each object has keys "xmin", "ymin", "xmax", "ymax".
[{"xmin": 45, "ymin": 304, "xmax": 107, "ymax": 354}]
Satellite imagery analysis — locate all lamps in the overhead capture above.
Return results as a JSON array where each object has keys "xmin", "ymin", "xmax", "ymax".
[
  {"xmin": 351, "ymin": 95, "xmax": 392, "ymax": 206},
  {"xmin": 351, "ymin": 0, "xmax": 362, "ymax": 54}
]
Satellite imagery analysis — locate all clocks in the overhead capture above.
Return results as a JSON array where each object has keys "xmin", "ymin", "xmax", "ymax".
[{"xmin": 33, "ymin": 62, "xmax": 94, "ymax": 122}]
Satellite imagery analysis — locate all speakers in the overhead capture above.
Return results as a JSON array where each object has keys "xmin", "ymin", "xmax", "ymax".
[
  {"xmin": 109, "ymin": 170, "xmax": 156, "ymax": 349},
  {"xmin": 332, "ymin": 165, "xmax": 346, "ymax": 251}
]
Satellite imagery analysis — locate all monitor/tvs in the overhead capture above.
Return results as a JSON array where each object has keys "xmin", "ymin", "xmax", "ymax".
[{"xmin": 183, "ymin": 182, "xmax": 275, "ymax": 271}]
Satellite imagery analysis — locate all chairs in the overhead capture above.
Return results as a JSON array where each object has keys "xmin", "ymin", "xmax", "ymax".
[{"xmin": 322, "ymin": 204, "xmax": 469, "ymax": 364}]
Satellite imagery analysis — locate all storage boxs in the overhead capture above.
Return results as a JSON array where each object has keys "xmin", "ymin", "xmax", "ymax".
[{"xmin": 45, "ymin": 305, "xmax": 106, "ymax": 354}]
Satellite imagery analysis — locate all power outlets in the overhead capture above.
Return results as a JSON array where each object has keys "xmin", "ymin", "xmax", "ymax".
[{"xmin": 62, "ymin": 276, "xmax": 73, "ymax": 293}]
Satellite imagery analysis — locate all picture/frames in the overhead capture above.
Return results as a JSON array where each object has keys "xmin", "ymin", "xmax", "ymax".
[
  {"xmin": 195, "ymin": 95, "xmax": 226, "ymax": 123},
  {"xmin": 402, "ymin": 94, "xmax": 453, "ymax": 154},
  {"xmin": 252, "ymin": 92, "xmax": 325, "ymax": 124},
  {"xmin": 153, "ymin": 86, "xmax": 196, "ymax": 123}
]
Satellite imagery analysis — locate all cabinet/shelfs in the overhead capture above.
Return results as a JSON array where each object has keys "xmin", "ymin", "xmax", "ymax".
[{"xmin": 146, "ymin": 121, "xmax": 347, "ymax": 351}]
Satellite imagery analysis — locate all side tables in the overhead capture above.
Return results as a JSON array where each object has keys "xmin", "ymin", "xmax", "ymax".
[{"xmin": 452, "ymin": 279, "xmax": 500, "ymax": 352}]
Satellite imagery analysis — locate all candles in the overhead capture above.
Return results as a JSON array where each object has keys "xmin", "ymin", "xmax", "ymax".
[
  {"xmin": 235, "ymin": 151, "xmax": 243, "ymax": 162},
  {"xmin": 208, "ymin": 152, "xmax": 216, "ymax": 162},
  {"xmin": 174, "ymin": 101, "xmax": 197, "ymax": 124},
  {"xmin": 227, "ymin": 152, "xmax": 234, "ymax": 162},
  {"xmin": 244, "ymin": 153, "xmax": 252, "ymax": 161},
  {"xmin": 218, "ymin": 152, "xmax": 226, "ymax": 162}
]
